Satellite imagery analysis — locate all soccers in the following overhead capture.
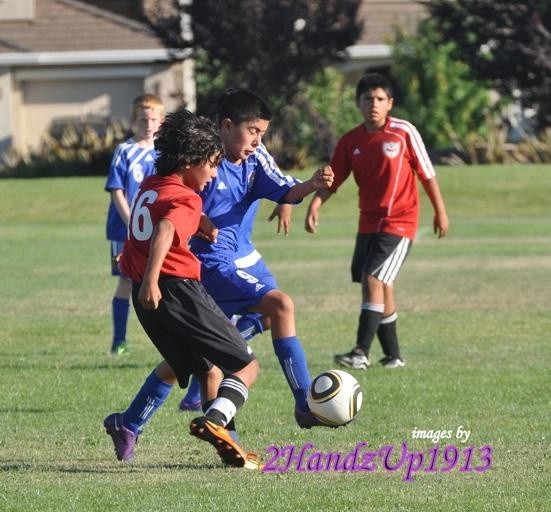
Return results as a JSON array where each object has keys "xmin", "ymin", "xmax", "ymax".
[{"xmin": 307, "ymin": 369, "xmax": 364, "ymax": 426}]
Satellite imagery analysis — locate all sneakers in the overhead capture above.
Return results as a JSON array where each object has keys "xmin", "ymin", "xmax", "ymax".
[
  {"xmin": 179, "ymin": 400, "xmax": 204, "ymax": 412},
  {"xmin": 334, "ymin": 347, "xmax": 372, "ymax": 371},
  {"xmin": 294, "ymin": 403, "xmax": 359, "ymax": 429},
  {"xmin": 104, "ymin": 412, "xmax": 143, "ymax": 462},
  {"xmin": 110, "ymin": 342, "xmax": 127, "ymax": 357},
  {"xmin": 190, "ymin": 416, "xmax": 248, "ymax": 467},
  {"xmin": 378, "ymin": 357, "xmax": 405, "ymax": 367}
]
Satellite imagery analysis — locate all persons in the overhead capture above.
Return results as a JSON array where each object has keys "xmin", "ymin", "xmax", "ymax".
[
  {"xmin": 121, "ymin": 109, "xmax": 261, "ymax": 469},
  {"xmin": 101, "ymin": 86, "xmax": 342, "ymax": 461},
  {"xmin": 305, "ymin": 73, "xmax": 450, "ymax": 370},
  {"xmin": 104, "ymin": 94, "xmax": 164, "ymax": 357},
  {"xmin": 179, "ymin": 198, "xmax": 293, "ymax": 411}
]
